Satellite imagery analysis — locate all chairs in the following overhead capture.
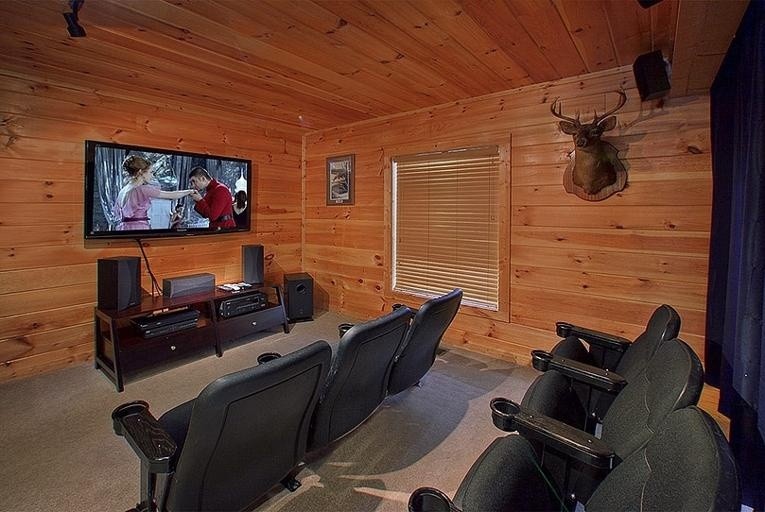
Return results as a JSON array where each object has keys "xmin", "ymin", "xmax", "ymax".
[
  {"xmin": 112, "ymin": 288, "xmax": 465, "ymax": 512},
  {"xmin": 407, "ymin": 301, "xmax": 739, "ymax": 512}
]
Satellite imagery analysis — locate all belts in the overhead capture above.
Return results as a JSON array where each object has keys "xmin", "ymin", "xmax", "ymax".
[{"xmin": 209, "ymin": 214, "xmax": 233, "ymax": 226}]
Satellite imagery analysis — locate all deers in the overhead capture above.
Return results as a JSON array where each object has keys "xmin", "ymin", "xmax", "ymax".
[{"xmin": 549, "ymin": 87, "xmax": 628, "ymax": 195}]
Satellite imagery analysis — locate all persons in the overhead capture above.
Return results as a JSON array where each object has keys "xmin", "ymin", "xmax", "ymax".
[{"xmin": 113, "ymin": 156, "xmax": 247, "ymax": 230}]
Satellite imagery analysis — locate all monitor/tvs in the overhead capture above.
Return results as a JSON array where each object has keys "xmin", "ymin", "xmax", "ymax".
[{"xmin": 85, "ymin": 139, "xmax": 252, "ymax": 239}]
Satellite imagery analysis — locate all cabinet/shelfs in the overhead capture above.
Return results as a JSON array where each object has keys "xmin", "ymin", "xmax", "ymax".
[
  {"xmin": 93, "ymin": 281, "xmax": 290, "ymax": 392},
  {"xmin": 62, "ymin": 0, "xmax": 87, "ymax": 37}
]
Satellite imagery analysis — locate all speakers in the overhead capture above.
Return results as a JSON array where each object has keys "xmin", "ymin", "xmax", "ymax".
[
  {"xmin": 98, "ymin": 256, "xmax": 142, "ymax": 312},
  {"xmin": 632, "ymin": 49, "xmax": 671, "ymax": 102},
  {"xmin": 284, "ymin": 272, "xmax": 313, "ymax": 322},
  {"xmin": 242, "ymin": 244, "xmax": 264, "ymax": 284}
]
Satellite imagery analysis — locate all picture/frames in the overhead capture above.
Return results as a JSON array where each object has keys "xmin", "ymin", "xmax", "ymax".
[{"xmin": 326, "ymin": 154, "xmax": 354, "ymax": 205}]
